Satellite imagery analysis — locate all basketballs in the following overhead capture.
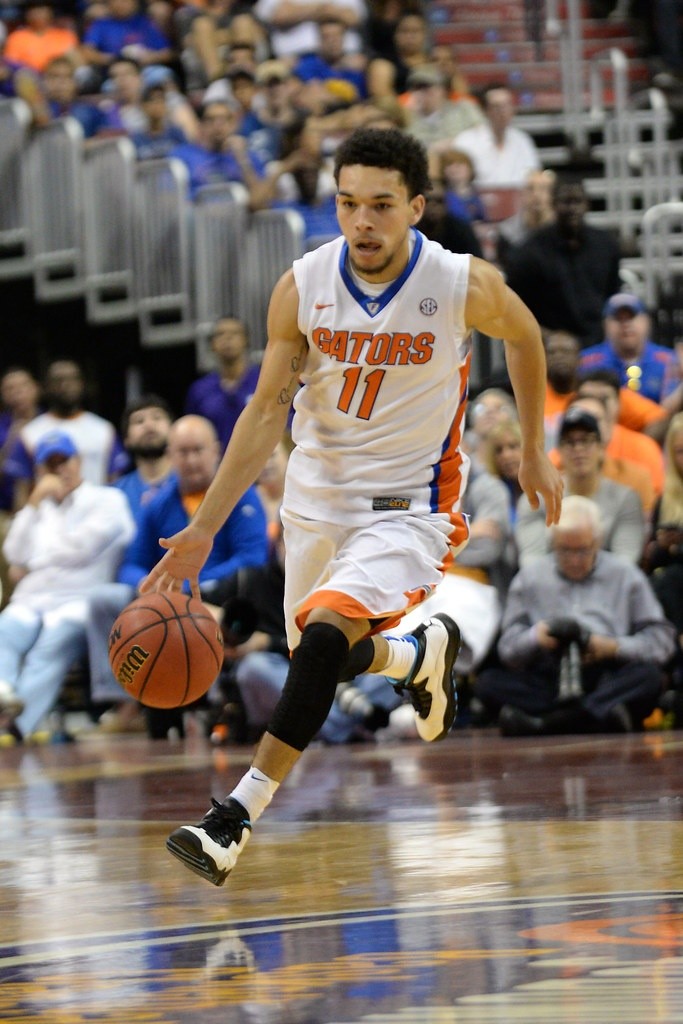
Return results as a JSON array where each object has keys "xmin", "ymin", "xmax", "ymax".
[{"xmin": 108, "ymin": 592, "xmax": 225, "ymax": 708}]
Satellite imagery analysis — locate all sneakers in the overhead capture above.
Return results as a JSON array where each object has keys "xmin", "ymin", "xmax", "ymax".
[
  {"xmin": 386, "ymin": 613, "xmax": 460, "ymax": 743},
  {"xmin": 168, "ymin": 796, "xmax": 250, "ymax": 886}
]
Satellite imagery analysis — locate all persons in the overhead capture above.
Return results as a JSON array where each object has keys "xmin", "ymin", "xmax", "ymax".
[
  {"xmin": 139, "ymin": 128, "xmax": 564, "ymax": 886},
  {"xmin": 0, "ymin": 0, "xmax": 682, "ymax": 748}
]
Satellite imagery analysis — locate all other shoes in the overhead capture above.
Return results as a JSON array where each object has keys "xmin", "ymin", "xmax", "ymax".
[
  {"xmin": 603, "ymin": 702, "xmax": 635, "ymax": 734},
  {"xmin": 498, "ymin": 706, "xmax": 541, "ymax": 737}
]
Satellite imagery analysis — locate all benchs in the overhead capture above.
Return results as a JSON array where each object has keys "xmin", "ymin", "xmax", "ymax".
[{"xmin": 408, "ymin": 0, "xmax": 672, "ymax": 113}]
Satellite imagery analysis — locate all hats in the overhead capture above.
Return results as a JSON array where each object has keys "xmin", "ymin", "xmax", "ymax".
[
  {"xmin": 605, "ymin": 293, "xmax": 641, "ymax": 315},
  {"xmin": 560, "ymin": 407, "xmax": 602, "ymax": 444},
  {"xmin": 35, "ymin": 432, "xmax": 76, "ymax": 464}
]
